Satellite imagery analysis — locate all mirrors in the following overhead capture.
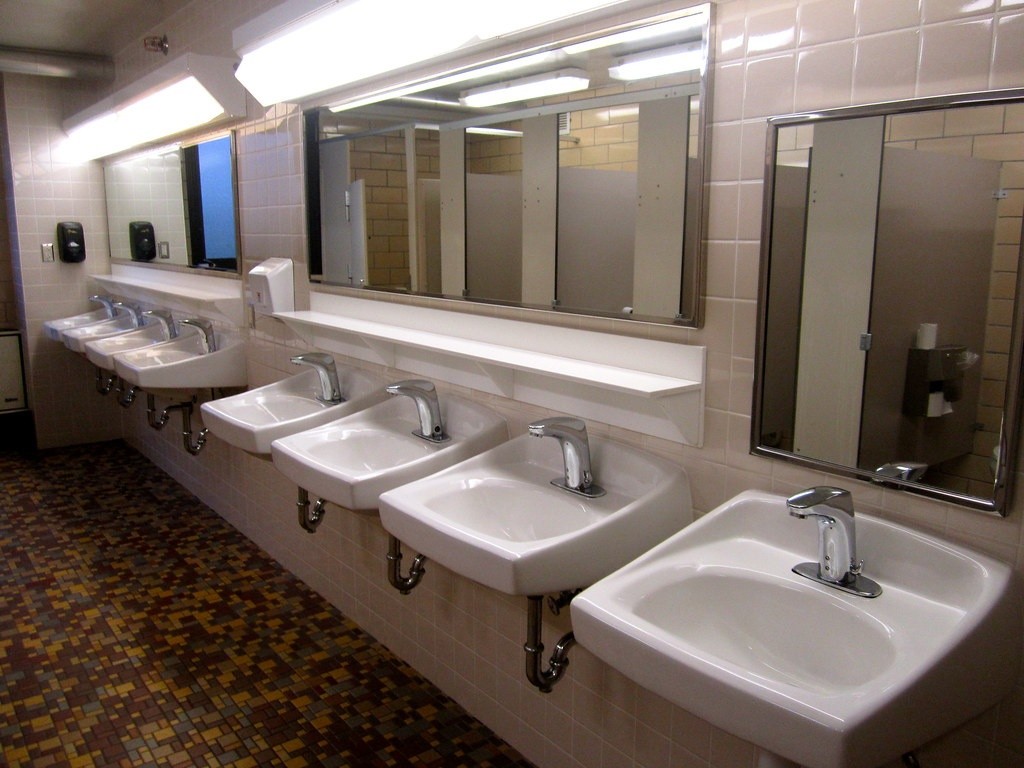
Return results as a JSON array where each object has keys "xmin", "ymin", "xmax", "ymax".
[
  {"xmin": 100, "ymin": 130, "xmax": 246, "ymax": 280},
  {"xmin": 297, "ymin": 0, "xmax": 717, "ymax": 331},
  {"xmin": 745, "ymin": 84, "xmax": 1024, "ymax": 522}
]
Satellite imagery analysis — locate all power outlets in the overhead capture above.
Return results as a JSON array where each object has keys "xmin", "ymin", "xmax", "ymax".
[
  {"xmin": 41, "ymin": 243, "xmax": 55, "ymax": 263},
  {"xmin": 158, "ymin": 241, "xmax": 169, "ymax": 259}
]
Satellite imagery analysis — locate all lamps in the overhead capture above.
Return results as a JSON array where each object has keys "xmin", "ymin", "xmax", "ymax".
[
  {"xmin": 459, "ymin": 67, "xmax": 593, "ymax": 110},
  {"xmin": 231, "ymin": 0, "xmax": 625, "ymax": 109},
  {"xmin": 144, "ymin": 32, "xmax": 169, "ymax": 54},
  {"xmin": 61, "ymin": 52, "xmax": 243, "ymax": 163},
  {"xmin": 607, "ymin": 41, "xmax": 702, "ymax": 82}
]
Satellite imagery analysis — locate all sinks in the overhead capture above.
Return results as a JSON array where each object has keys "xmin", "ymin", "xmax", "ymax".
[
  {"xmin": 570, "ymin": 487, "xmax": 1024, "ymax": 767},
  {"xmin": 111, "ymin": 330, "xmax": 248, "ymax": 389},
  {"xmin": 200, "ymin": 369, "xmax": 393, "ymax": 462},
  {"xmin": 378, "ymin": 426, "xmax": 693, "ymax": 596},
  {"xmin": 271, "ymin": 388, "xmax": 509, "ymax": 511},
  {"xmin": 41, "ymin": 307, "xmax": 198, "ymax": 372}
]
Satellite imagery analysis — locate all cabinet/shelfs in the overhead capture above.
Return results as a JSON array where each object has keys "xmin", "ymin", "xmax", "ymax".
[{"xmin": 0, "ymin": 328, "xmax": 30, "ymax": 414}]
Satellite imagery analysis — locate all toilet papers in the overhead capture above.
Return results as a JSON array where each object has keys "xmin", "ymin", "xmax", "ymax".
[
  {"xmin": 915, "ymin": 323, "xmax": 938, "ymax": 349},
  {"xmin": 927, "ymin": 392, "xmax": 953, "ymax": 418}
]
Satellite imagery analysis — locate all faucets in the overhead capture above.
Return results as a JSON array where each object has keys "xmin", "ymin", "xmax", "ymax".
[
  {"xmin": 289, "ymin": 353, "xmax": 347, "ymax": 405},
  {"xmin": 112, "ymin": 301, "xmax": 144, "ymax": 328},
  {"xmin": 873, "ymin": 460, "xmax": 929, "ymax": 483},
  {"xmin": 176, "ymin": 318, "xmax": 216, "ymax": 355},
  {"xmin": 141, "ymin": 310, "xmax": 178, "ymax": 340},
  {"xmin": 786, "ymin": 486, "xmax": 884, "ymax": 600},
  {"xmin": 385, "ymin": 379, "xmax": 451, "ymax": 443},
  {"xmin": 86, "ymin": 294, "xmax": 119, "ymax": 319},
  {"xmin": 528, "ymin": 416, "xmax": 607, "ymax": 498}
]
